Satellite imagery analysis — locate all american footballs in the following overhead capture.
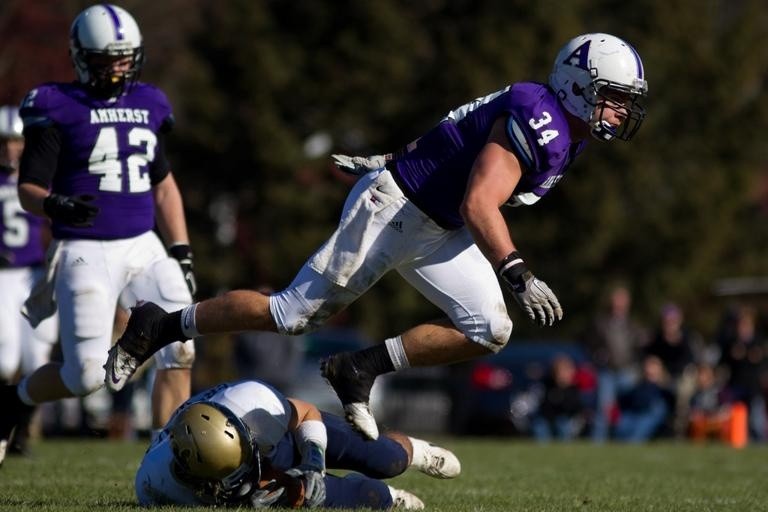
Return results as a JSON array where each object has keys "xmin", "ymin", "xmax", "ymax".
[{"xmin": 260, "ymin": 478, "xmax": 305, "ymax": 509}]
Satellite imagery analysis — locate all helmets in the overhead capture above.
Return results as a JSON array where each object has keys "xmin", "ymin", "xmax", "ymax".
[
  {"xmin": 67, "ymin": 3, "xmax": 145, "ymax": 98},
  {"xmin": 551, "ymin": 30, "xmax": 647, "ymax": 144},
  {"xmin": 0, "ymin": 106, "xmax": 26, "ymax": 170},
  {"xmin": 170, "ymin": 401, "xmax": 260, "ymax": 504}
]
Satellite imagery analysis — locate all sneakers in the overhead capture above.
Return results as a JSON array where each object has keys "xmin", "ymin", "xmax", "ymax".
[
  {"xmin": 408, "ymin": 433, "xmax": 461, "ymax": 480},
  {"xmin": 388, "ymin": 486, "xmax": 424, "ymax": 511},
  {"xmin": 318, "ymin": 350, "xmax": 381, "ymax": 441},
  {"xmin": 102, "ymin": 300, "xmax": 168, "ymax": 392}
]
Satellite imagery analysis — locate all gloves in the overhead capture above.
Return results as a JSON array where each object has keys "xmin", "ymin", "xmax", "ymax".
[
  {"xmin": 238, "ymin": 477, "xmax": 284, "ymax": 511},
  {"xmin": 286, "ymin": 467, "xmax": 326, "ymax": 508},
  {"xmin": 42, "ymin": 194, "xmax": 100, "ymax": 229},
  {"xmin": 494, "ymin": 251, "xmax": 563, "ymax": 328},
  {"xmin": 167, "ymin": 241, "xmax": 198, "ymax": 297},
  {"xmin": 333, "ymin": 149, "xmax": 393, "ymax": 174}
]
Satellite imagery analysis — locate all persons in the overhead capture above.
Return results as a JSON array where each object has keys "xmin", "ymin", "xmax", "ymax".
[
  {"xmin": 135, "ymin": 377, "xmax": 461, "ymax": 511},
  {"xmin": 0, "ymin": 4, "xmax": 196, "ymax": 468},
  {"xmin": 106, "ymin": 32, "xmax": 649, "ymax": 442},
  {"xmin": 0, "ymin": 106, "xmax": 60, "ymax": 453}
]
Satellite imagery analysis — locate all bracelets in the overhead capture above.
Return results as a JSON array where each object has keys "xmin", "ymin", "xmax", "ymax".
[
  {"xmin": 496, "ymin": 251, "xmax": 529, "ymax": 284},
  {"xmin": 43, "ymin": 193, "xmax": 58, "ymax": 218},
  {"xmin": 167, "ymin": 242, "xmax": 193, "ymax": 265}
]
